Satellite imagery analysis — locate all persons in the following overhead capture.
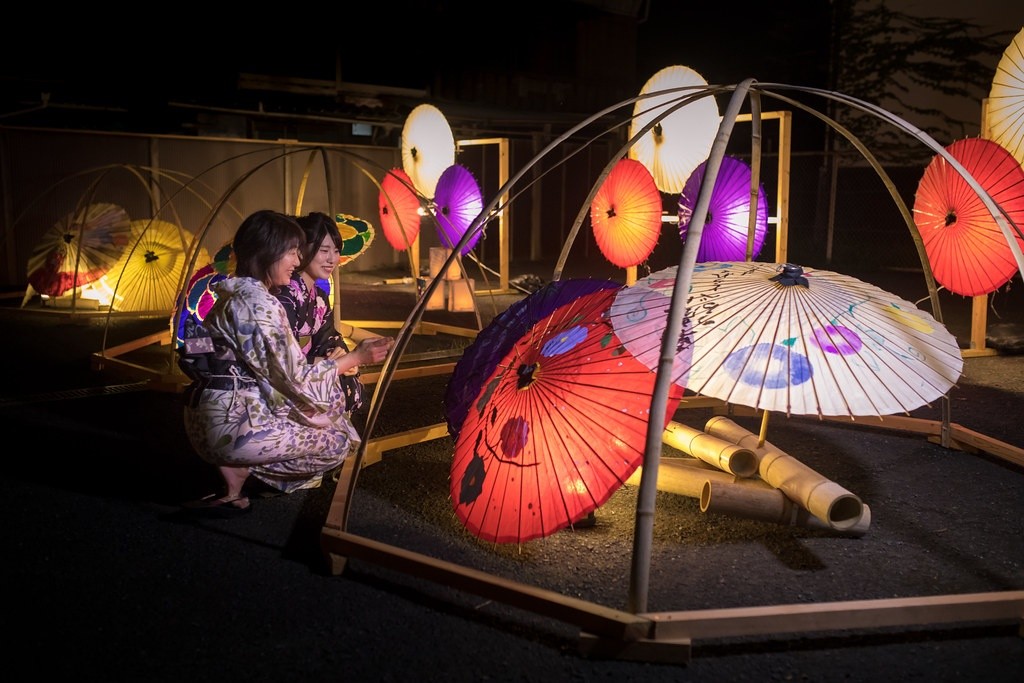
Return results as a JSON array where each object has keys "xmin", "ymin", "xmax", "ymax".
[{"xmin": 177, "ymin": 209, "xmax": 395, "ymax": 518}]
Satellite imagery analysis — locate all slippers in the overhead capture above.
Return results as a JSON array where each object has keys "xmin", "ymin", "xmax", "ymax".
[{"xmin": 184, "ymin": 491, "xmax": 250, "ymax": 512}]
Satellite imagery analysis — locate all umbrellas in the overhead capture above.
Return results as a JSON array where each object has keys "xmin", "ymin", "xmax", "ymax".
[
  {"xmin": 914, "ymin": 135, "xmax": 1024, "ymax": 299},
  {"xmin": 631, "ymin": 65, "xmax": 723, "ymax": 198},
  {"xmin": 23, "ymin": 201, "xmax": 209, "ymax": 315},
  {"xmin": 982, "ymin": 29, "xmax": 1024, "ymax": 172},
  {"xmin": 379, "ymin": 104, "xmax": 484, "ymax": 256},
  {"xmin": 443, "ymin": 261, "xmax": 965, "ymax": 546},
  {"xmin": 677, "ymin": 156, "xmax": 768, "ymax": 262},
  {"xmin": 592, "ymin": 160, "xmax": 665, "ymax": 272}
]
